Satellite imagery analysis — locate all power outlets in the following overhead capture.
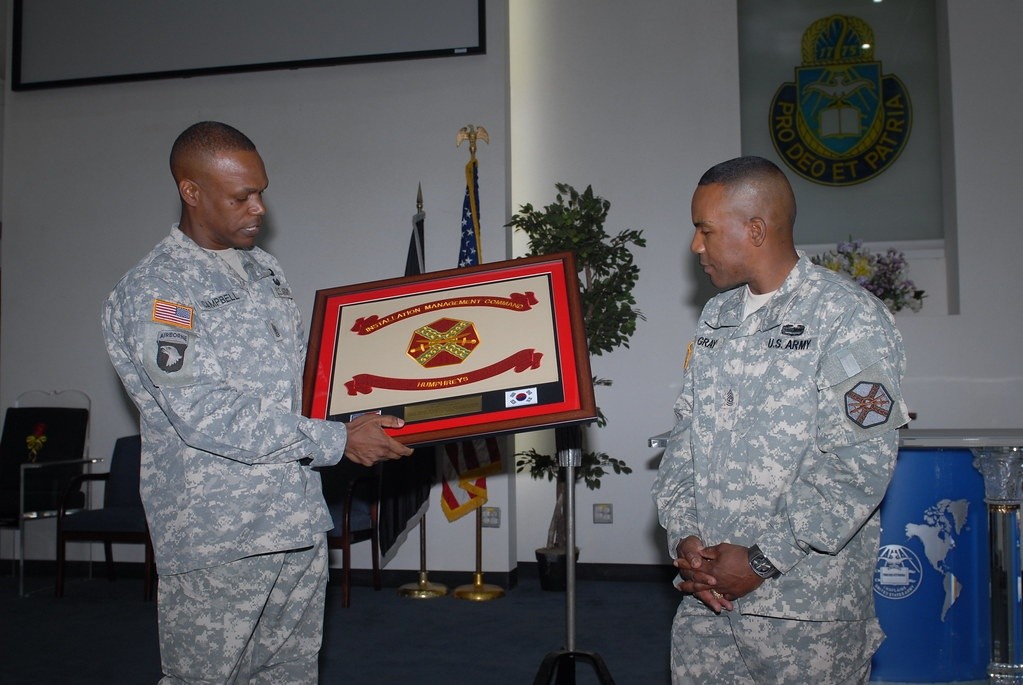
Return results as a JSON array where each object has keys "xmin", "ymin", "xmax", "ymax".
[
  {"xmin": 593, "ymin": 503, "xmax": 613, "ymax": 524},
  {"xmin": 481, "ymin": 507, "xmax": 502, "ymax": 528}
]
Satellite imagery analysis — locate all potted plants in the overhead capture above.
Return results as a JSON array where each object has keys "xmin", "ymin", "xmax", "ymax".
[{"xmin": 503, "ymin": 183, "xmax": 645, "ymax": 592}]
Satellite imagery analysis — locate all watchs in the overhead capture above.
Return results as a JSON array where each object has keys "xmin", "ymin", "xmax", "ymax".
[{"xmin": 748, "ymin": 543, "xmax": 782, "ymax": 580}]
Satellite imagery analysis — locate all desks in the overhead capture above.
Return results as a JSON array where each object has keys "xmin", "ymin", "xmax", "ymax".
[{"xmin": 648, "ymin": 428, "xmax": 1023, "ymax": 685}]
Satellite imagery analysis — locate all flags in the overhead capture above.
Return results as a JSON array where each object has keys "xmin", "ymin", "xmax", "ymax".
[
  {"xmin": 441, "ymin": 160, "xmax": 500, "ymax": 521},
  {"xmin": 377, "ymin": 211, "xmax": 431, "ymax": 568}
]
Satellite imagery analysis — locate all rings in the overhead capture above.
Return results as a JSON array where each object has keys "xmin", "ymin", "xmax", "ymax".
[{"xmin": 711, "ymin": 588, "xmax": 723, "ymax": 599}]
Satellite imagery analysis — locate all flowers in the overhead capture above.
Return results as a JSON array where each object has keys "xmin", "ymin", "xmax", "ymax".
[{"xmin": 810, "ymin": 234, "xmax": 925, "ymax": 313}]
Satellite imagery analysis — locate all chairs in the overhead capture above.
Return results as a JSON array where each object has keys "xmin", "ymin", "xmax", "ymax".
[
  {"xmin": 309, "ymin": 457, "xmax": 381, "ymax": 608},
  {"xmin": 0, "ymin": 389, "xmax": 154, "ymax": 601}
]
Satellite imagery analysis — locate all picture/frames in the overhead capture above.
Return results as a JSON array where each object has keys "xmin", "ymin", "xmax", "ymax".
[{"xmin": 301, "ymin": 251, "xmax": 597, "ymax": 446}]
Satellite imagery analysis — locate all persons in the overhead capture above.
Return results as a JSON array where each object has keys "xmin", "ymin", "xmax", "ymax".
[
  {"xmin": 102, "ymin": 120, "xmax": 415, "ymax": 685},
  {"xmin": 651, "ymin": 156, "xmax": 911, "ymax": 685}
]
